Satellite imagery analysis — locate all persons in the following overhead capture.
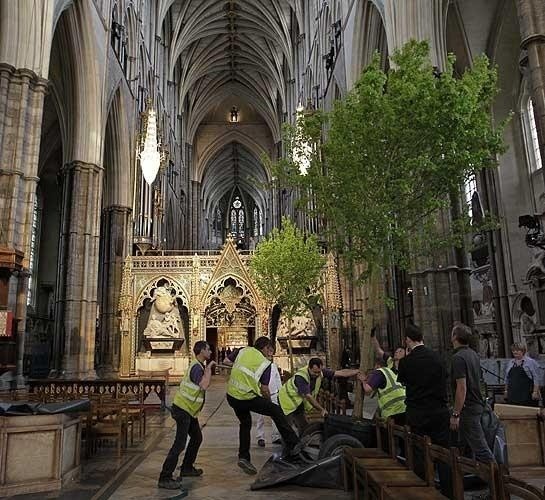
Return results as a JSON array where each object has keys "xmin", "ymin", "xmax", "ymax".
[
  {"xmin": 277, "ymin": 358, "xmax": 359, "ymax": 447},
  {"xmin": 223, "ymin": 336, "xmax": 300, "ymax": 475},
  {"xmin": 359, "ymin": 324, "xmax": 542, "ymax": 500},
  {"xmin": 256, "ymin": 348, "xmax": 282, "ymax": 447},
  {"xmin": 218, "ymin": 347, "xmax": 232, "ymax": 363},
  {"xmin": 158, "ymin": 341, "xmax": 217, "ymax": 489}
]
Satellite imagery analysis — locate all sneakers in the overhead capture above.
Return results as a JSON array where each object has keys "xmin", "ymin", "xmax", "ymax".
[
  {"xmin": 288, "ymin": 435, "xmax": 311, "ymax": 456},
  {"xmin": 158, "ymin": 478, "xmax": 180, "ymax": 489},
  {"xmin": 472, "ymin": 487, "xmax": 492, "ymax": 500},
  {"xmin": 396, "ymin": 453, "xmax": 406, "ymax": 465},
  {"xmin": 237, "ymin": 458, "xmax": 257, "ymax": 475},
  {"xmin": 180, "ymin": 468, "xmax": 202, "ymax": 477}
]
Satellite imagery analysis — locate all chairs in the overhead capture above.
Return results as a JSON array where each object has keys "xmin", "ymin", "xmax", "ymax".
[
  {"xmin": 302, "ymin": 391, "xmax": 347, "ymax": 428},
  {"xmin": 344, "ymin": 417, "xmax": 544, "ymax": 500},
  {"xmin": 0, "ymin": 386, "xmax": 148, "ymax": 459}
]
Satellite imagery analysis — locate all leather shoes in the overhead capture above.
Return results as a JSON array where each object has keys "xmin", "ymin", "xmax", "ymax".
[
  {"xmin": 257, "ymin": 440, "xmax": 264, "ymax": 447},
  {"xmin": 271, "ymin": 438, "xmax": 282, "ymax": 444}
]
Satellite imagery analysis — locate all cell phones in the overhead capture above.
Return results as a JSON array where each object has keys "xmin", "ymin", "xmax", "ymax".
[{"xmin": 370, "ymin": 327, "xmax": 376, "ymax": 338}]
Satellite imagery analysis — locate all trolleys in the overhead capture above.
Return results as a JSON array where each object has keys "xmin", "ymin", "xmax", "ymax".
[{"xmin": 297, "ymin": 412, "xmax": 399, "ymax": 480}]
